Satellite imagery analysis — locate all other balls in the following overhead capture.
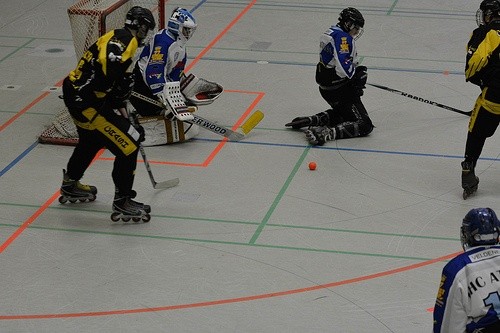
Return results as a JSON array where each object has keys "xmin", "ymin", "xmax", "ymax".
[{"xmin": 308, "ymin": 162, "xmax": 317, "ymax": 170}]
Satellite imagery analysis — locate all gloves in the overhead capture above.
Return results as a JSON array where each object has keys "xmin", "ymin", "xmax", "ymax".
[
  {"xmin": 128, "ymin": 113, "xmax": 146, "ymax": 142},
  {"xmin": 351, "ymin": 66, "xmax": 368, "ymax": 86}
]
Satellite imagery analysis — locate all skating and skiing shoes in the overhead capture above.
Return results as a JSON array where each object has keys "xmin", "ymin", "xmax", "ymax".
[
  {"xmin": 58, "ymin": 169, "xmax": 97, "ymax": 204},
  {"xmin": 304, "ymin": 125, "xmax": 336, "ymax": 146},
  {"xmin": 461, "ymin": 161, "xmax": 479, "ymax": 200},
  {"xmin": 285, "ymin": 111, "xmax": 330, "ymax": 129},
  {"xmin": 110, "ymin": 185, "xmax": 151, "ymax": 223}
]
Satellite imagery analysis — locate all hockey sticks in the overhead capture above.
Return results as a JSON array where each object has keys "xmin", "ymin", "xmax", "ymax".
[
  {"xmin": 173, "ymin": 109, "xmax": 264, "ymax": 141},
  {"xmin": 366, "ymin": 81, "xmax": 476, "ymax": 118},
  {"xmin": 121, "ymin": 105, "xmax": 180, "ymax": 189}
]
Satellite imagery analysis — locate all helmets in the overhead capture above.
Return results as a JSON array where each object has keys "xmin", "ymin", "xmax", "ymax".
[
  {"xmin": 336, "ymin": 7, "xmax": 365, "ymax": 41},
  {"xmin": 475, "ymin": 0, "xmax": 500, "ymax": 27},
  {"xmin": 459, "ymin": 208, "xmax": 500, "ymax": 251},
  {"xmin": 125, "ymin": 6, "xmax": 156, "ymax": 48},
  {"xmin": 167, "ymin": 7, "xmax": 199, "ymax": 46}
]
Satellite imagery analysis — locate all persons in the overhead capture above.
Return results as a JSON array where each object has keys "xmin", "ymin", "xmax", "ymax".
[
  {"xmin": 432, "ymin": 208, "xmax": 500, "ymax": 333},
  {"xmin": 58, "ymin": 6, "xmax": 156, "ymax": 224},
  {"xmin": 285, "ymin": 7, "xmax": 374, "ymax": 147},
  {"xmin": 461, "ymin": 0, "xmax": 500, "ymax": 200},
  {"xmin": 127, "ymin": 7, "xmax": 223, "ymax": 146}
]
